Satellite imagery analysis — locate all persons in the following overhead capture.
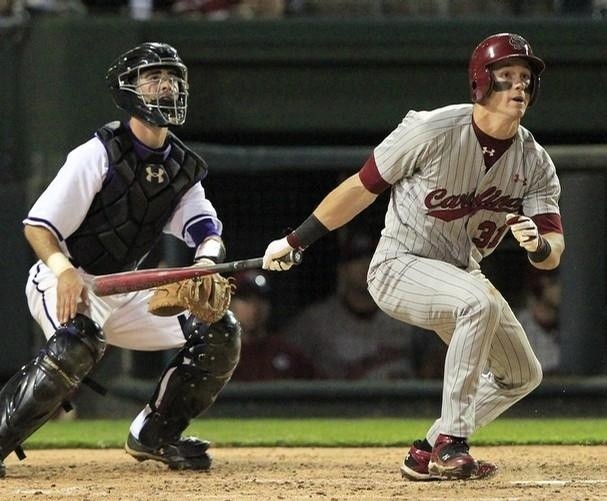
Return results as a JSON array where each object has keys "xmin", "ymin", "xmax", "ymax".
[
  {"xmin": 226, "ymin": 268, "xmax": 322, "ymax": 382},
  {"xmin": 513, "ymin": 272, "xmax": 564, "ymax": 371},
  {"xmin": 281, "ymin": 229, "xmax": 418, "ymax": 381},
  {"xmin": 261, "ymin": 32, "xmax": 567, "ymax": 483},
  {"xmin": 0, "ymin": 42, "xmax": 244, "ymax": 481}
]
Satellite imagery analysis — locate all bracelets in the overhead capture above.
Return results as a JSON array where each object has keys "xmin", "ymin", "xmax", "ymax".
[
  {"xmin": 294, "ymin": 213, "xmax": 330, "ymax": 246},
  {"xmin": 528, "ymin": 236, "xmax": 552, "ymax": 263},
  {"xmin": 46, "ymin": 251, "xmax": 75, "ymax": 278}
]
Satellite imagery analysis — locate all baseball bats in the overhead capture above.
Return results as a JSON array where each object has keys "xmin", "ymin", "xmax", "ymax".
[{"xmin": 91, "ymin": 250, "xmax": 302, "ymax": 297}]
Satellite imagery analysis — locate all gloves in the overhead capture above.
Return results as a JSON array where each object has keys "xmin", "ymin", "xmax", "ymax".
[
  {"xmin": 506, "ymin": 214, "xmax": 538, "ymax": 252},
  {"xmin": 262, "ymin": 236, "xmax": 295, "ymax": 271}
]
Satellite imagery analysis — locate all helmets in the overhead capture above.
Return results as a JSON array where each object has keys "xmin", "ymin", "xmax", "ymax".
[
  {"xmin": 469, "ymin": 33, "xmax": 545, "ymax": 107},
  {"xmin": 105, "ymin": 43, "xmax": 189, "ymax": 127}
]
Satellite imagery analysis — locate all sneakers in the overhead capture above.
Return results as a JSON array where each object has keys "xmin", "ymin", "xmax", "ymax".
[
  {"xmin": 125, "ymin": 433, "xmax": 210, "ymax": 470},
  {"xmin": 428, "ymin": 434, "xmax": 474, "ymax": 476},
  {"xmin": 401, "ymin": 439, "xmax": 497, "ymax": 480}
]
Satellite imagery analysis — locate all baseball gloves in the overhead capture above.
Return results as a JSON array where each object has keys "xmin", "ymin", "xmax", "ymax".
[{"xmin": 147, "ymin": 262, "xmax": 237, "ymax": 322}]
